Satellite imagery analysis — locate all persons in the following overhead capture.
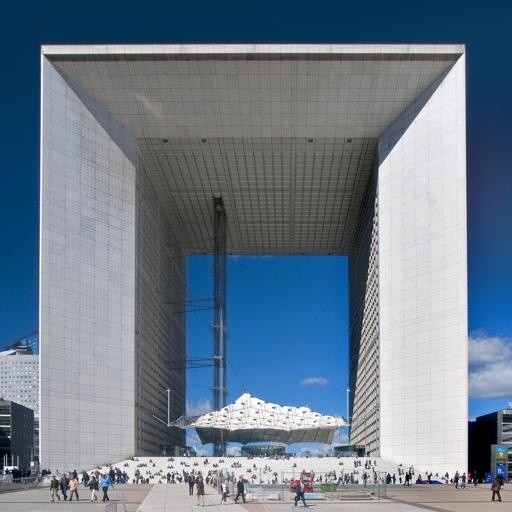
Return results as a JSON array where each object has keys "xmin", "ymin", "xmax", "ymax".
[
  {"xmin": 187, "ymin": 471, "xmax": 195, "ymax": 496},
  {"xmin": 219, "ymin": 479, "xmax": 228, "ymax": 503},
  {"xmin": 234, "ymin": 476, "xmax": 248, "ymax": 504},
  {"xmin": 196, "ymin": 478, "xmax": 205, "ymax": 507},
  {"xmin": 115, "ymin": 451, "xmax": 479, "ymax": 489},
  {"xmin": 48, "ymin": 462, "xmax": 115, "ymax": 504},
  {"xmin": 0, "ymin": 465, "xmax": 62, "ymax": 482},
  {"xmin": 490, "ymin": 473, "xmax": 504, "ymax": 501},
  {"xmin": 294, "ymin": 478, "xmax": 308, "ymax": 506}
]
